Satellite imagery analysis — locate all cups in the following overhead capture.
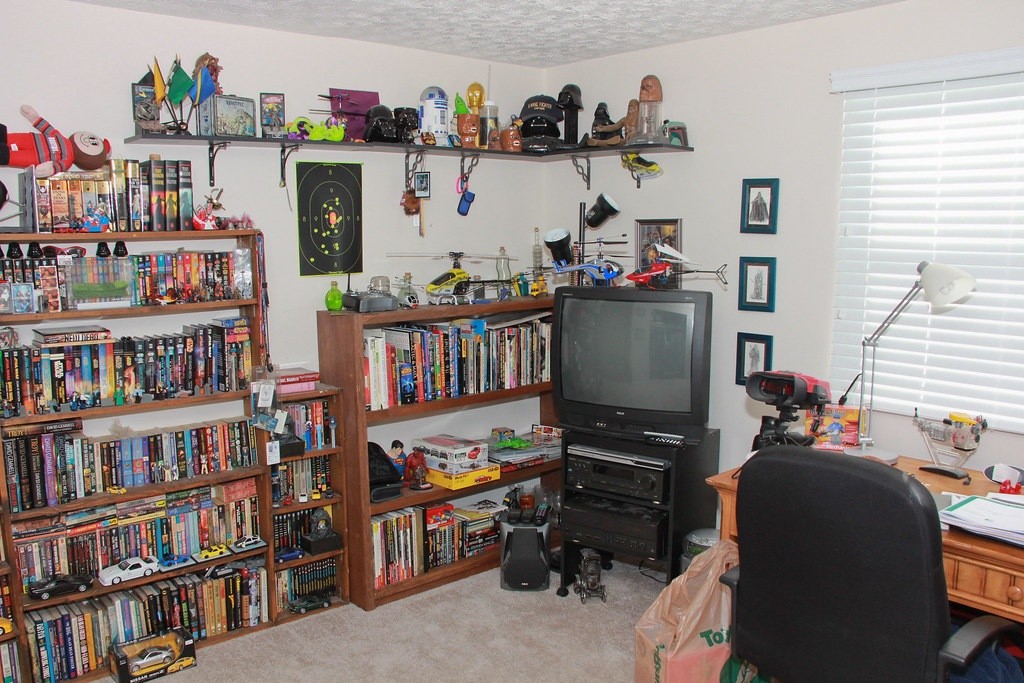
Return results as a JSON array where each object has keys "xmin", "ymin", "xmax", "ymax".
[{"xmin": 508, "ymin": 483, "xmax": 561, "ymax": 530}]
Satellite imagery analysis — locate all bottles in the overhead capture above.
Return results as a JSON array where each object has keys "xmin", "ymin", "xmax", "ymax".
[
  {"xmin": 517, "ymin": 273, "xmax": 528, "ymax": 296},
  {"xmin": 398, "ymin": 273, "xmax": 419, "ymax": 310},
  {"xmin": 496, "ymin": 246, "xmax": 511, "ymax": 300},
  {"xmin": 325, "ymin": 281, "xmax": 343, "ymax": 311},
  {"xmin": 477, "ymin": 100, "xmax": 499, "ymax": 150}
]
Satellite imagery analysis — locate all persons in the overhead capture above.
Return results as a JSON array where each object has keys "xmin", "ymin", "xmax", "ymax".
[
  {"xmin": 387, "ymin": 441, "xmax": 430, "ymax": 486},
  {"xmin": 581, "ymin": 98, "xmax": 639, "ymax": 147}
]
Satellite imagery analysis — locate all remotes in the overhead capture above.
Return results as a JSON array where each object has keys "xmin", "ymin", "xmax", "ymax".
[{"xmin": 501, "ymin": 504, "xmax": 552, "ymax": 526}]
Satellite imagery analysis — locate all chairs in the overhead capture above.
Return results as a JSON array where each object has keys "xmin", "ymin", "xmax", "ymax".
[{"xmin": 720, "ymin": 445, "xmax": 1018, "ymax": 683}]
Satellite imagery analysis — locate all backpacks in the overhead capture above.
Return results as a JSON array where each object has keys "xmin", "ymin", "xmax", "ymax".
[{"xmin": 500, "ymin": 528, "xmax": 549, "ymax": 592}]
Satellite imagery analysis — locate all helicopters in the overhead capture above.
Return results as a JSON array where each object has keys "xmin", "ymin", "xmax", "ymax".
[
  {"xmin": 552, "ymin": 233, "xmax": 635, "ymax": 287},
  {"xmin": 386, "ymin": 250, "xmax": 519, "ymax": 306},
  {"xmin": 525, "ymin": 258, "xmax": 556, "ymax": 299},
  {"xmin": 624, "ymin": 232, "xmax": 729, "ymax": 290}
]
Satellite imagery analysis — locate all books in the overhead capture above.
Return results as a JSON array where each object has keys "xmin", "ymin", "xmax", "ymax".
[
  {"xmin": 273, "ymin": 504, "xmax": 332, "ymax": 554},
  {"xmin": 0, "ymin": 316, "xmax": 252, "ymax": 419},
  {"xmin": 17, "ymin": 159, "xmax": 193, "ymax": 235},
  {"xmin": 0, "ymin": 529, "xmax": 22, "ymax": 683},
  {"xmin": 371, "ymin": 499, "xmax": 510, "ymax": 590},
  {"xmin": 24, "ymin": 557, "xmax": 268, "ymax": 683},
  {"xmin": 474, "ymin": 429, "xmax": 562, "ymax": 472},
  {"xmin": 282, "ymin": 398, "xmax": 331, "ymax": 447},
  {"xmin": 361, "ymin": 311, "xmax": 552, "ymax": 411},
  {"xmin": 271, "ymin": 556, "xmax": 335, "ymax": 613},
  {"xmin": 931, "ymin": 491, "xmax": 1024, "ymax": 548},
  {"xmin": 1, "ymin": 417, "xmax": 258, "ymax": 514},
  {"xmin": 269, "ymin": 367, "xmax": 320, "ymax": 394},
  {"xmin": 0, "ymin": 249, "xmax": 253, "ymax": 315},
  {"xmin": 1, "ymin": 479, "xmax": 260, "ymax": 594},
  {"xmin": 271, "ymin": 454, "xmax": 332, "ymax": 503}
]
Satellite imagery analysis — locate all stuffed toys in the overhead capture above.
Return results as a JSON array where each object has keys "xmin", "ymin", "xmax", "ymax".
[{"xmin": 0, "ymin": 104, "xmax": 112, "ymax": 179}]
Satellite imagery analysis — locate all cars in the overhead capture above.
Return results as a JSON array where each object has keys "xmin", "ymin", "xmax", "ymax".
[
  {"xmin": 106, "ymin": 485, "xmax": 128, "ymax": 495},
  {"xmin": 233, "ymin": 534, "xmax": 261, "ymax": 549},
  {"xmin": 98, "ymin": 555, "xmax": 160, "ymax": 587},
  {"xmin": 272, "ymin": 488, "xmax": 334, "ymax": 509},
  {"xmin": 0, "ymin": 617, "xmax": 13, "ymax": 635},
  {"xmin": 128, "ymin": 645, "xmax": 176, "ymax": 673},
  {"xmin": 27, "ymin": 573, "xmax": 94, "ymax": 601},
  {"xmin": 161, "ymin": 552, "xmax": 191, "ymax": 567},
  {"xmin": 198, "ymin": 544, "xmax": 227, "ymax": 559}
]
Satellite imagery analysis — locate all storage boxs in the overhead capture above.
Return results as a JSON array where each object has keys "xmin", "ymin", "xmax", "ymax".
[
  {"xmin": 196, "ymin": 93, "xmax": 255, "ymax": 140},
  {"xmin": 108, "ymin": 624, "xmax": 197, "ymax": 683},
  {"xmin": 634, "ymin": 622, "xmax": 782, "ymax": 683},
  {"xmin": 413, "ymin": 434, "xmax": 501, "ymax": 490},
  {"xmin": 491, "ymin": 427, "xmax": 515, "ymax": 441},
  {"xmin": 421, "ymin": 502, "xmax": 455, "ymax": 531},
  {"xmin": 805, "ymin": 404, "xmax": 866, "ymax": 448},
  {"xmin": 532, "ymin": 424, "xmax": 566, "ymax": 438}
]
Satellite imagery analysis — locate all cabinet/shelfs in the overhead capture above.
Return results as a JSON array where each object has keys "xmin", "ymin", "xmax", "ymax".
[
  {"xmin": 316, "ymin": 291, "xmax": 568, "ymax": 615},
  {"xmin": 557, "ymin": 426, "xmax": 720, "ymax": 597},
  {"xmin": 0, "ymin": 227, "xmax": 349, "ymax": 683}
]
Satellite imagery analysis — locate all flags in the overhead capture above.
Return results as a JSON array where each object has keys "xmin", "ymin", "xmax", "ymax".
[{"xmin": 139, "ymin": 61, "xmax": 217, "ymax": 106}]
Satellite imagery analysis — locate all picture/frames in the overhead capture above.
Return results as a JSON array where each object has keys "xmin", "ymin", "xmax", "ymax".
[
  {"xmin": 131, "ymin": 83, "xmax": 161, "ymax": 121},
  {"xmin": 736, "ymin": 332, "xmax": 773, "ymax": 385},
  {"xmin": 740, "ymin": 178, "xmax": 779, "ymax": 234},
  {"xmin": 414, "ymin": 172, "xmax": 430, "ymax": 198},
  {"xmin": 260, "ymin": 92, "xmax": 285, "ymax": 126},
  {"xmin": 738, "ymin": 257, "xmax": 776, "ymax": 313},
  {"xmin": 634, "ymin": 218, "xmax": 682, "ymax": 290}
]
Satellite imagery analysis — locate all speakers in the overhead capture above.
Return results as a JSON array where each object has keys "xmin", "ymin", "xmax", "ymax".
[{"xmin": 497, "ymin": 512, "xmax": 552, "ymax": 592}]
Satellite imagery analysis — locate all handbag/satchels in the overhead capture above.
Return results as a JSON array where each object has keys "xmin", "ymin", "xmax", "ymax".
[
  {"xmin": 367, "ymin": 442, "xmax": 403, "ymax": 502},
  {"xmin": 634, "ymin": 538, "xmax": 738, "ymax": 683}
]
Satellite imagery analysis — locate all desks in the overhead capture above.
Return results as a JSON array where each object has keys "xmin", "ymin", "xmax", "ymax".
[{"xmin": 705, "ymin": 455, "xmax": 1024, "ymax": 644}]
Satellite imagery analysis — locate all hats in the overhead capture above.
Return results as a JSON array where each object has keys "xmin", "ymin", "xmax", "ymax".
[{"xmin": 520, "ymin": 93, "xmax": 563, "ymax": 124}]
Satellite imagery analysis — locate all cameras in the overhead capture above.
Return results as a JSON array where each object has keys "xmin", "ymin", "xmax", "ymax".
[{"xmin": 549, "ymin": 286, "xmax": 713, "ymax": 440}]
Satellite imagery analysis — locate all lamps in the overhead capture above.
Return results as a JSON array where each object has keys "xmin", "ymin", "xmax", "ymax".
[
  {"xmin": 843, "ymin": 260, "xmax": 978, "ymax": 466},
  {"xmin": 543, "ymin": 191, "xmax": 621, "ymax": 286}
]
